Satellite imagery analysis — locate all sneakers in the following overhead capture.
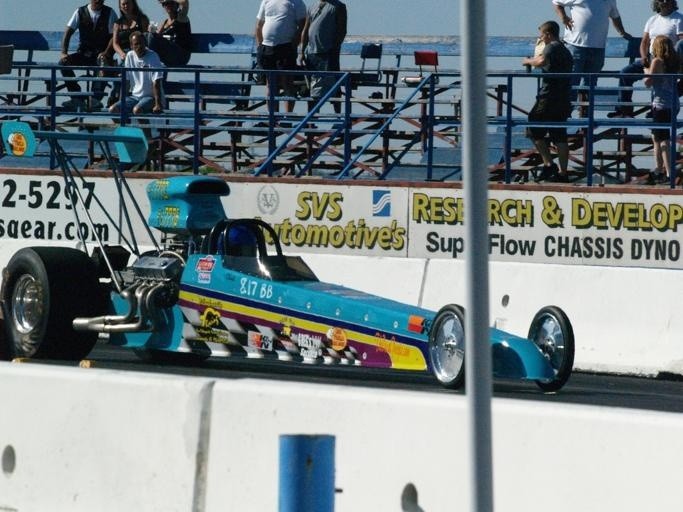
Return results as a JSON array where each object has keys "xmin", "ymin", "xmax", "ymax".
[
  {"xmin": 91, "ymin": 98, "xmax": 104, "ymax": 108},
  {"xmin": 533, "ymin": 162, "xmax": 559, "ymax": 182},
  {"xmin": 666, "ymin": 174, "xmax": 674, "ymax": 185},
  {"xmin": 606, "ymin": 112, "xmax": 633, "ymax": 118},
  {"xmin": 62, "ymin": 98, "xmax": 86, "ymax": 108},
  {"xmin": 651, "ymin": 171, "xmax": 665, "ymax": 183}
]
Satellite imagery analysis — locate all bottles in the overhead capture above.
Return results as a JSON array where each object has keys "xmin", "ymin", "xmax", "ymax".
[
  {"xmin": 642, "ymin": 57, "xmax": 650, "ymax": 75},
  {"xmin": 526, "ymin": 56, "xmax": 532, "ymax": 73}
]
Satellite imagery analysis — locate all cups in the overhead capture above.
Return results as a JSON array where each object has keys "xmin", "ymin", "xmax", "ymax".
[{"xmin": 149, "ymin": 20, "xmax": 158, "ymax": 34}]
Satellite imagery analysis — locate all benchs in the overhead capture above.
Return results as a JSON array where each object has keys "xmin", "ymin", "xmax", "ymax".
[{"xmin": 0, "ymin": 30, "xmax": 683, "ymax": 186}]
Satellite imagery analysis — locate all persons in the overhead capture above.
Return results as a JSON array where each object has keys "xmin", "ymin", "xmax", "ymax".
[
  {"xmin": 552, "ymin": 0, "xmax": 633, "ymax": 134},
  {"xmin": 296, "ymin": 0, "xmax": 347, "ymax": 128},
  {"xmin": 643, "ymin": 35, "xmax": 681, "ymax": 182},
  {"xmin": 255, "ymin": 0, "xmax": 306, "ymax": 128},
  {"xmin": 522, "ymin": 21, "xmax": 573, "ymax": 183},
  {"xmin": 58, "ymin": 0, "xmax": 192, "ymax": 138},
  {"xmin": 607, "ymin": 0, "xmax": 683, "ymax": 118}
]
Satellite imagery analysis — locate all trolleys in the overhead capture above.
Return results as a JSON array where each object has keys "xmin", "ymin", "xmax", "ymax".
[
  {"xmin": 337, "ymin": 42, "xmax": 382, "ymax": 83},
  {"xmin": 401, "ymin": 50, "xmax": 439, "ymax": 87}
]
